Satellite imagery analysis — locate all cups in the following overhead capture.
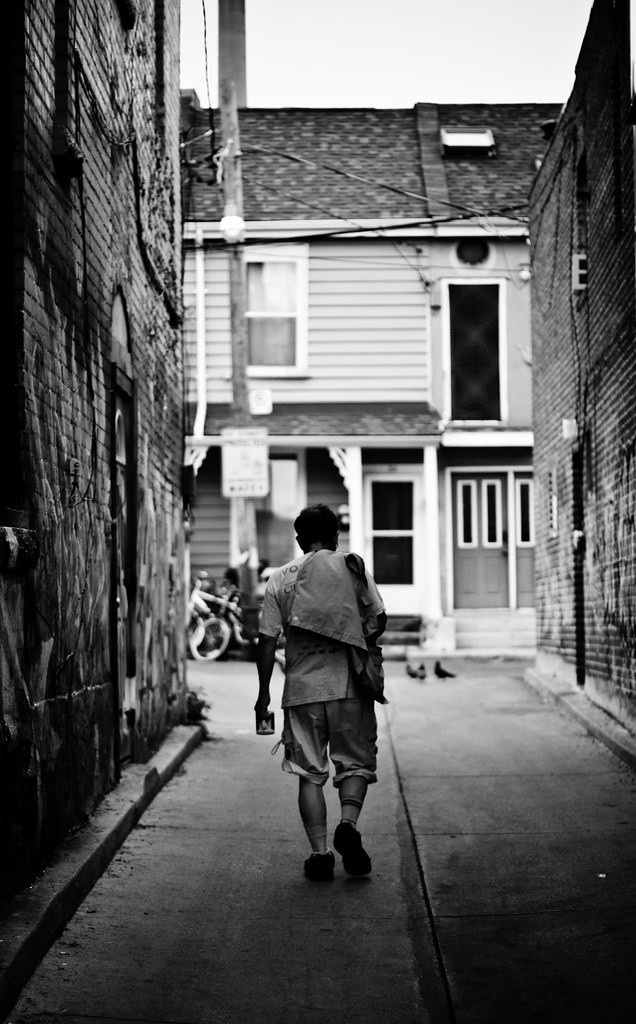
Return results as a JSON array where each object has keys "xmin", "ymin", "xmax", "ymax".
[{"xmin": 255, "ymin": 711, "xmax": 275, "ymax": 735}]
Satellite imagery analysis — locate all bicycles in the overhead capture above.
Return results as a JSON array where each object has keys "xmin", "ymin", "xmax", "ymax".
[{"xmin": 187, "ymin": 573, "xmax": 283, "ymax": 661}]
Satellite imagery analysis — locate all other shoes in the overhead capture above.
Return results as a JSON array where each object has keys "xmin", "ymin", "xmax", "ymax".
[
  {"xmin": 333, "ymin": 822, "xmax": 372, "ymax": 875},
  {"xmin": 304, "ymin": 850, "xmax": 335, "ymax": 881}
]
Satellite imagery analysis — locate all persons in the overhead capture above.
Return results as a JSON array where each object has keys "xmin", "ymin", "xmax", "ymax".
[{"xmin": 252, "ymin": 503, "xmax": 389, "ymax": 882}]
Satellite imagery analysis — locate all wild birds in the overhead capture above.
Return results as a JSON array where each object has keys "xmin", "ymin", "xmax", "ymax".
[{"xmin": 405, "ymin": 660, "xmax": 456, "ymax": 684}]
